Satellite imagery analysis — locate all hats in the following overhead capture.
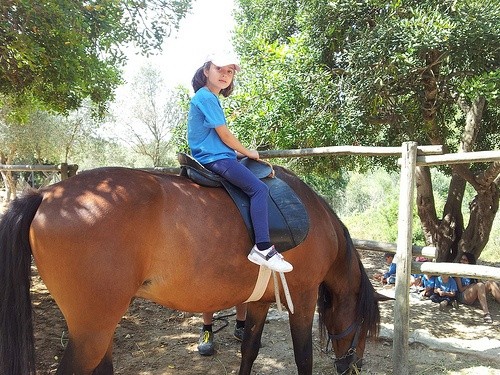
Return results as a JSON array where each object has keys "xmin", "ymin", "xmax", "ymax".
[{"xmin": 203, "ymin": 48, "xmax": 242, "ymax": 73}]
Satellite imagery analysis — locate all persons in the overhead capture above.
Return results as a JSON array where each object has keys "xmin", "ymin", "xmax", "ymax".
[
  {"xmin": 453, "ymin": 252, "xmax": 500, "ymax": 323},
  {"xmin": 197, "ymin": 303, "xmax": 248, "ymax": 356},
  {"xmin": 410, "ymin": 257, "xmax": 435, "ymax": 297},
  {"xmin": 188, "ymin": 50, "xmax": 294, "ymax": 273},
  {"xmin": 430, "ymin": 276, "xmax": 458, "ymax": 309},
  {"xmin": 373, "ymin": 252, "xmax": 397, "ymax": 283}
]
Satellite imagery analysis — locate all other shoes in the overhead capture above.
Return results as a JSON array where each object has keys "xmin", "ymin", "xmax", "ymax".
[
  {"xmin": 483, "ymin": 312, "xmax": 493, "ymax": 323},
  {"xmin": 451, "ymin": 300, "xmax": 458, "ymax": 308}
]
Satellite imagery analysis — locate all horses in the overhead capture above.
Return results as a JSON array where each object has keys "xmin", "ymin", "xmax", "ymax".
[{"xmin": 0, "ymin": 166, "xmax": 397, "ymax": 375}]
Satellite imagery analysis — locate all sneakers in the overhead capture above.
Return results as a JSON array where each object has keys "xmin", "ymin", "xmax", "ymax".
[
  {"xmin": 198, "ymin": 330, "xmax": 214, "ymax": 355},
  {"xmin": 233, "ymin": 323, "xmax": 246, "ymax": 341},
  {"xmin": 247, "ymin": 244, "xmax": 293, "ymax": 272}
]
